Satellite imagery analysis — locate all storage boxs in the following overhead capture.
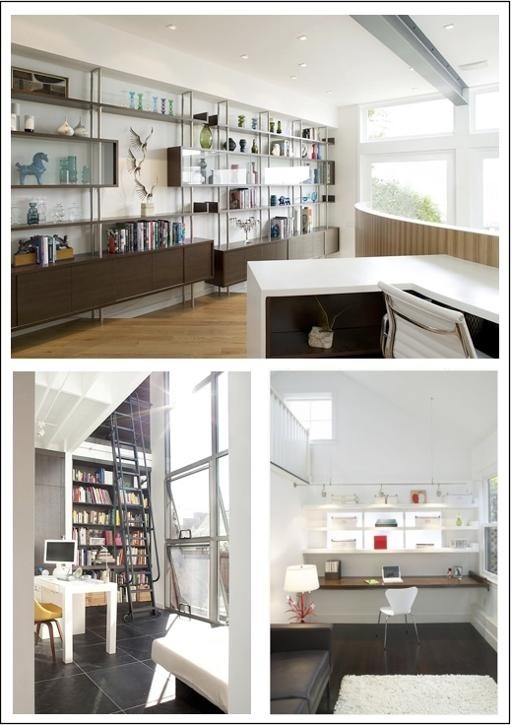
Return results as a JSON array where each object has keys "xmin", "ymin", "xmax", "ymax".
[
  {"xmin": 137, "ymin": 585, "xmax": 151, "ymax": 602},
  {"xmin": 86, "ymin": 592, "xmax": 107, "ymax": 607}
]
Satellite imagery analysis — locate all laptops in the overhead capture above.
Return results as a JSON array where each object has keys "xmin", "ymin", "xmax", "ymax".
[{"xmin": 382, "ymin": 565, "xmax": 403, "ymax": 583}]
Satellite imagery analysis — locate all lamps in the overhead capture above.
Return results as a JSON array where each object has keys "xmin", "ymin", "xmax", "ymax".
[{"xmin": 284, "ymin": 564, "xmax": 320, "ymax": 622}]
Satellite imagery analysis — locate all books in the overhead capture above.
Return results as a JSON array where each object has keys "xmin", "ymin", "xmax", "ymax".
[
  {"xmin": 272, "ymin": 217, "xmax": 294, "ymax": 239},
  {"xmin": 120, "ymin": 490, "xmax": 148, "ymax": 507},
  {"xmin": 230, "ymin": 188, "xmax": 257, "ymax": 209},
  {"xmin": 73, "ymin": 528, "xmax": 113, "ymax": 546},
  {"xmin": 125, "ymin": 530, "xmax": 145, "ymax": 545},
  {"xmin": 117, "ymin": 587, "xmax": 138, "ymax": 604},
  {"xmin": 106, "ymin": 221, "xmax": 185, "ymax": 254},
  {"xmin": 116, "ymin": 547, "xmax": 146, "ymax": 566},
  {"xmin": 117, "ymin": 510, "xmax": 149, "ymax": 526},
  {"xmin": 74, "ymin": 510, "xmax": 110, "ymax": 524},
  {"xmin": 73, "ymin": 488, "xmax": 112, "ymax": 505},
  {"xmin": 74, "ymin": 465, "xmax": 114, "ymax": 484}
]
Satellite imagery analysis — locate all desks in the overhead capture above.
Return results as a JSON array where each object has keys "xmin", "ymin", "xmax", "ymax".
[
  {"xmin": 247, "ymin": 255, "xmax": 499, "ymax": 358},
  {"xmin": 320, "ymin": 575, "xmax": 487, "ymax": 624},
  {"xmin": 37, "ymin": 574, "xmax": 119, "ymax": 664},
  {"xmin": 150, "ymin": 627, "xmax": 229, "ymax": 714}
]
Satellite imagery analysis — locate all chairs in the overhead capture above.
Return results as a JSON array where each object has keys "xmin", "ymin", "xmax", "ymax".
[
  {"xmin": 34, "ymin": 598, "xmax": 65, "ymax": 664},
  {"xmin": 377, "ymin": 281, "xmax": 479, "ymax": 359},
  {"xmin": 378, "ymin": 587, "xmax": 422, "ymax": 652}
]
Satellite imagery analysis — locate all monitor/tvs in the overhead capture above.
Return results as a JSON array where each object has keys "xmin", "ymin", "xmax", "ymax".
[{"xmin": 44, "ymin": 539, "xmax": 77, "ymax": 568}]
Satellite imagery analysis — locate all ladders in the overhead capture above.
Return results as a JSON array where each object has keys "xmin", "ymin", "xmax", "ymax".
[{"xmin": 109, "ymin": 390, "xmax": 161, "ymax": 624}]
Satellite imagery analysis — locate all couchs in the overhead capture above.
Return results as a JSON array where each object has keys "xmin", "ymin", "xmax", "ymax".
[{"xmin": 270, "ymin": 623, "xmax": 334, "ymax": 714}]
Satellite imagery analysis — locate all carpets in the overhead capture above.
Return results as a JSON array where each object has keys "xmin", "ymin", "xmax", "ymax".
[{"xmin": 334, "ymin": 673, "xmax": 497, "ymax": 715}]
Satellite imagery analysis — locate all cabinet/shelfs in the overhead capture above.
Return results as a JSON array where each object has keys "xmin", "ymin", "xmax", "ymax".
[
  {"xmin": 301, "ymin": 505, "xmax": 479, "ymax": 575},
  {"xmin": 11, "ymin": 41, "xmax": 340, "ymax": 332},
  {"xmin": 73, "ymin": 455, "xmax": 152, "ymax": 586}
]
